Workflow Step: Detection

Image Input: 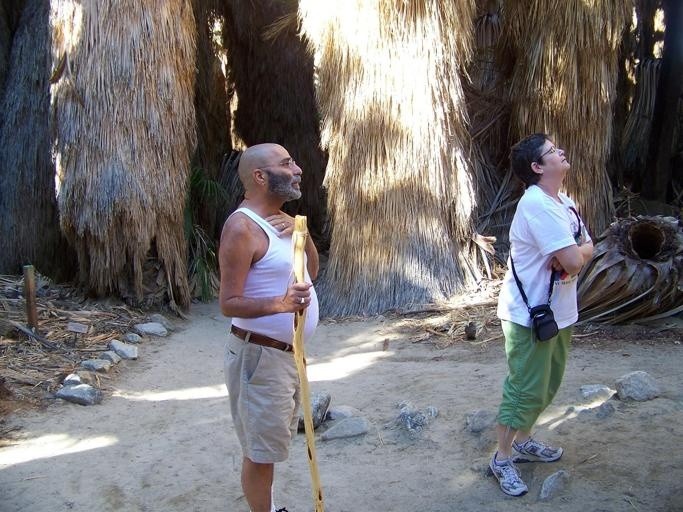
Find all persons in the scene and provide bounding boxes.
[488,131,595,497]
[216,143,320,511]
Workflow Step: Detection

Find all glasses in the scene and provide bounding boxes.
[256,160,295,170]
[537,145,555,161]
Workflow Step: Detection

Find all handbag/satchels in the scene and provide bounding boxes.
[530,305,558,341]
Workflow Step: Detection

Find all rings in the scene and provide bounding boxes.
[299,296,305,307]
[280,221,286,227]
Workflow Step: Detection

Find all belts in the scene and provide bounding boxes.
[230,324,293,352]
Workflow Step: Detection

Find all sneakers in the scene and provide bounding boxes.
[511,436,563,462]
[488,450,528,496]
